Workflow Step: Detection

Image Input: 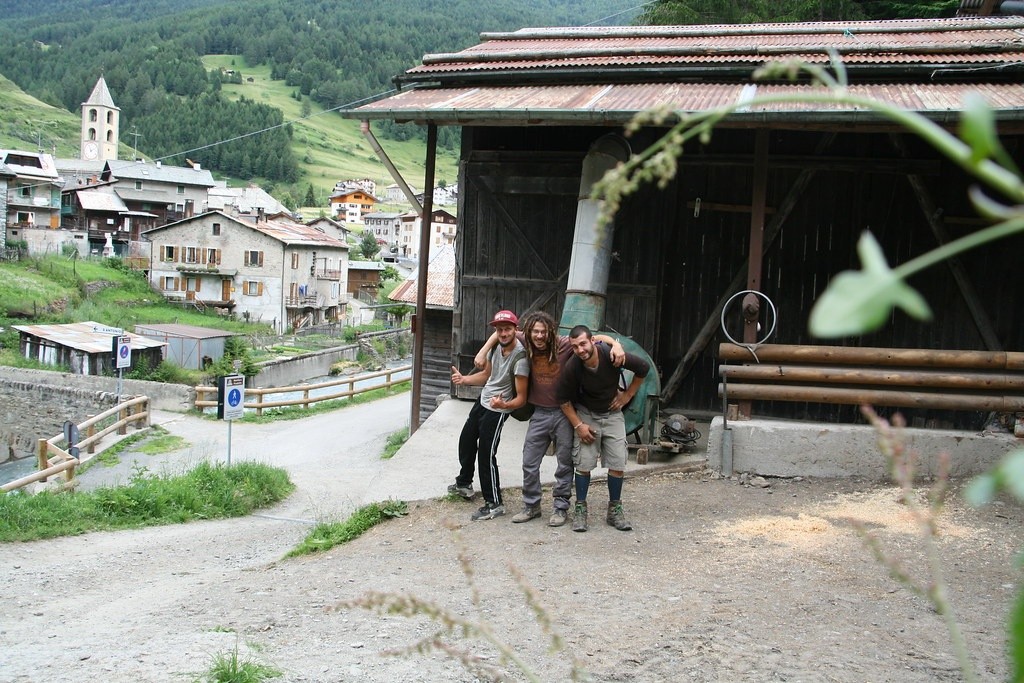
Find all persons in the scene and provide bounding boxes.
[447,310,650,532]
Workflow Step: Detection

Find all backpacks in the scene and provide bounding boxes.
[489,341,535,422]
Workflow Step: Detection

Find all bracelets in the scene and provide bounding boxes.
[574,422,583,429]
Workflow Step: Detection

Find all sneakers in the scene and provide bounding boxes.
[471,501,506,521]
[447,483,474,498]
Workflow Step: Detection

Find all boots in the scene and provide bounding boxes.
[572,501,587,531]
[606,500,632,531]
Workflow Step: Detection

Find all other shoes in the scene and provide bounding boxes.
[512,506,542,523]
[547,508,567,526]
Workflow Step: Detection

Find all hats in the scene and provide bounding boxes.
[489,310,519,326]
